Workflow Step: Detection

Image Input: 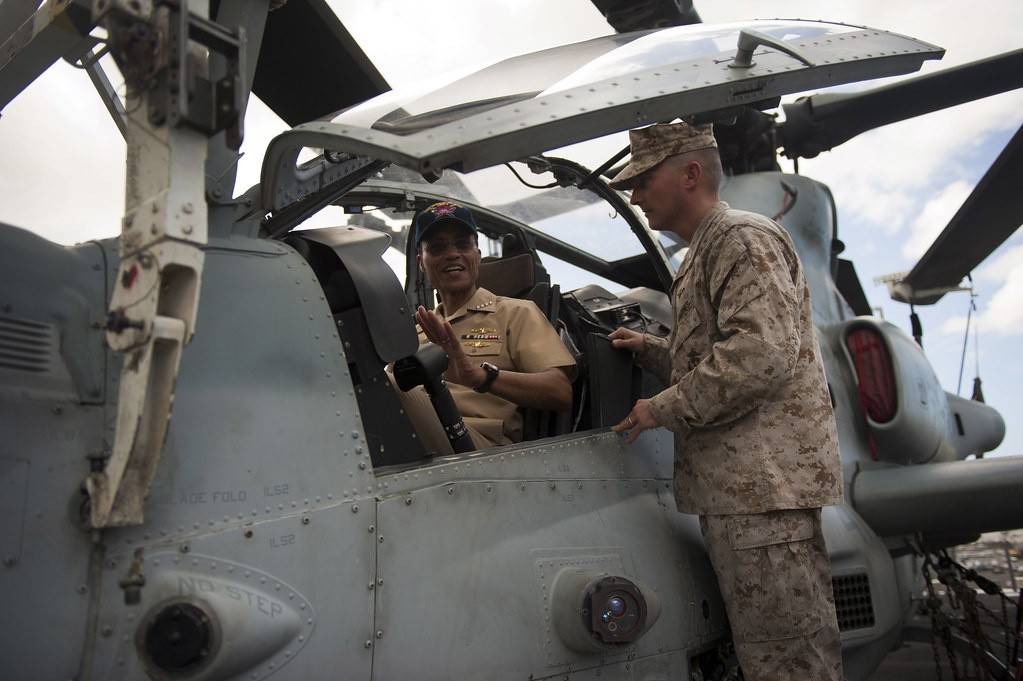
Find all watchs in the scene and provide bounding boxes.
[472,362,499,393]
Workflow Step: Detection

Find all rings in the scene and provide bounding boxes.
[628,418,634,426]
[442,338,450,343]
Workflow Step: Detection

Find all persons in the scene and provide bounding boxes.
[383,203,577,457]
[605,121,850,681]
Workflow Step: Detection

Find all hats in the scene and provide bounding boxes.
[609,121,717,191]
[416,202,477,247]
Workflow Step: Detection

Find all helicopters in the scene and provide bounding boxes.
[0,0,1023,681]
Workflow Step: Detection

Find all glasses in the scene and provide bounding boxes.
[420,240,478,256]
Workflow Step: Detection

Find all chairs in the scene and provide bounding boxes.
[476,252,589,441]
